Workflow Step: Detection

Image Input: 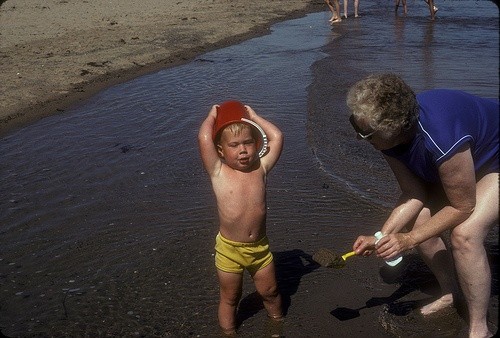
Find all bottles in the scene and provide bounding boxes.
[374,231,403,267]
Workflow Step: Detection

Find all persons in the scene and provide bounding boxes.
[347,70,500,338]
[196,101,285,338]
[325,0,439,25]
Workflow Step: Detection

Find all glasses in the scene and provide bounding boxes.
[349,114,380,140]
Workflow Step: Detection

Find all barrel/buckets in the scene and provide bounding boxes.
[212,100,268,161]
